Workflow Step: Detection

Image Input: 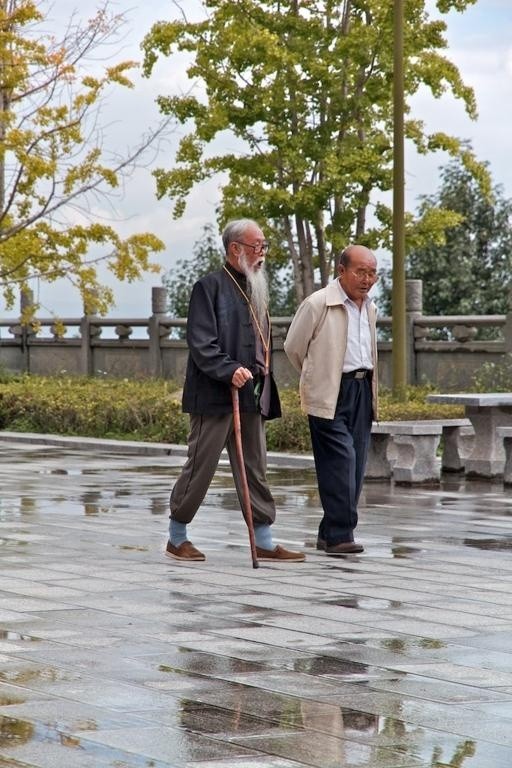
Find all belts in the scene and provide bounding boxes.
[343,371,373,379]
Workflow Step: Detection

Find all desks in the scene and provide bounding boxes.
[426,393,512,483]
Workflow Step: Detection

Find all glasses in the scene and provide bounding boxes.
[236,240,269,255]
[350,270,378,284]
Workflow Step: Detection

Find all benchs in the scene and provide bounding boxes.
[364,418,512,488]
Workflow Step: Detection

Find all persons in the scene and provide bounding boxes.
[165,221,309,562]
[284,245,380,555]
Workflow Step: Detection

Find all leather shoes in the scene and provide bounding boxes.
[318,537,365,554]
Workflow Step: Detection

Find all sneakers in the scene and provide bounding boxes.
[256,544,304,562]
[164,540,205,561]
